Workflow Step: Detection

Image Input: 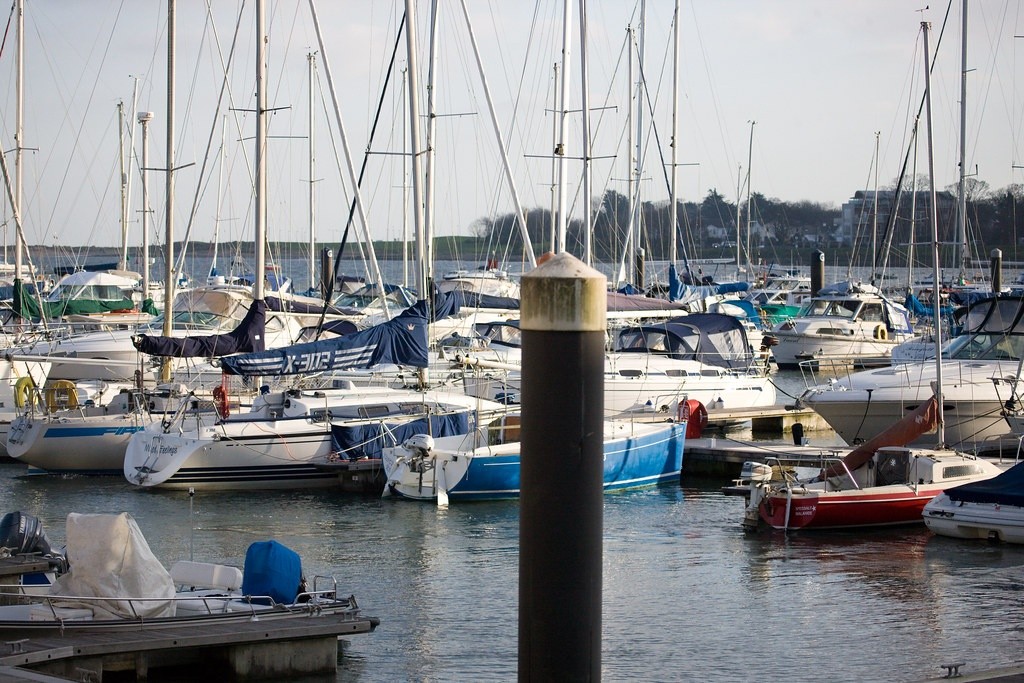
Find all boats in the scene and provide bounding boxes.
[2,510,353,624]
[721,445,1005,532]
[921,460,1024,547]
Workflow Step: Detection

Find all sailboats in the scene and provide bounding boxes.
[1,0,1024,498]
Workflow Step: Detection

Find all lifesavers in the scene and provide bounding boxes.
[42,376,81,414]
[11,375,39,410]
[875,324,889,340]
